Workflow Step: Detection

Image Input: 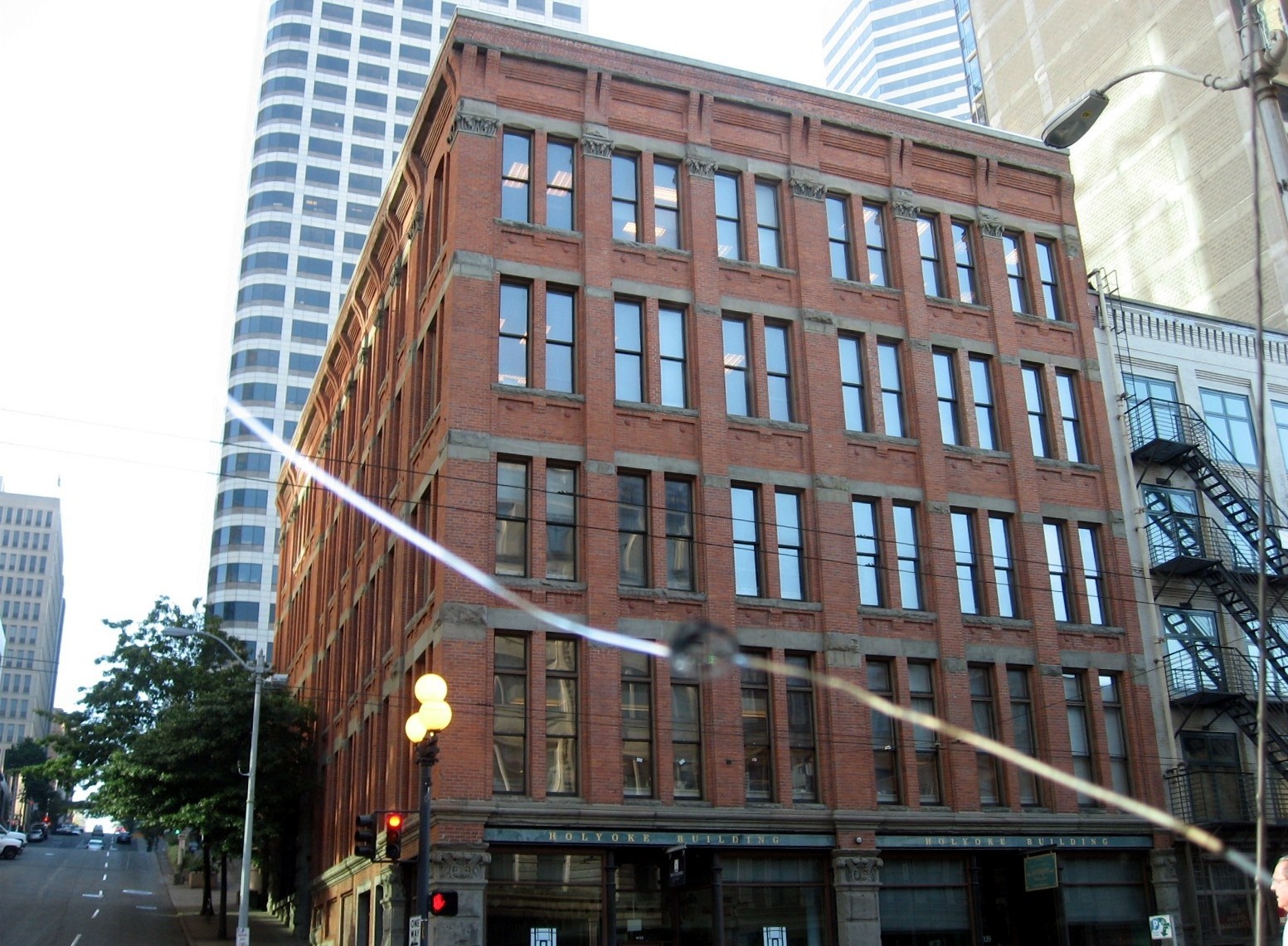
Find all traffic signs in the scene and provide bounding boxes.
[409,915,422,946]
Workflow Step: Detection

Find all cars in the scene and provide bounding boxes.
[56,824,84,835]
[85,839,104,851]
[29,830,43,841]
[116,832,131,843]
[92,827,103,836]
[0,825,28,859]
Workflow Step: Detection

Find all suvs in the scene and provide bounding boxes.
[32,823,47,839]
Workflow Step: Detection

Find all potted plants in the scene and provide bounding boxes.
[249,857,263,892]
[187,854,219,887]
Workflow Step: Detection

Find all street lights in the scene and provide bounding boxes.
[161,627,271,946]
[1037,1,1287,223]
[405,673,452,946]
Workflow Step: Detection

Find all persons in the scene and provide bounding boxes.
[189,839,198,853]
[1270,855,1288,946]
[147,838,154,852]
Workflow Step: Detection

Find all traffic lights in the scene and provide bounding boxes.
[354,815,377,859]
[430,889,459,917]
[386,814,402,859]
[173,828,181,836]
